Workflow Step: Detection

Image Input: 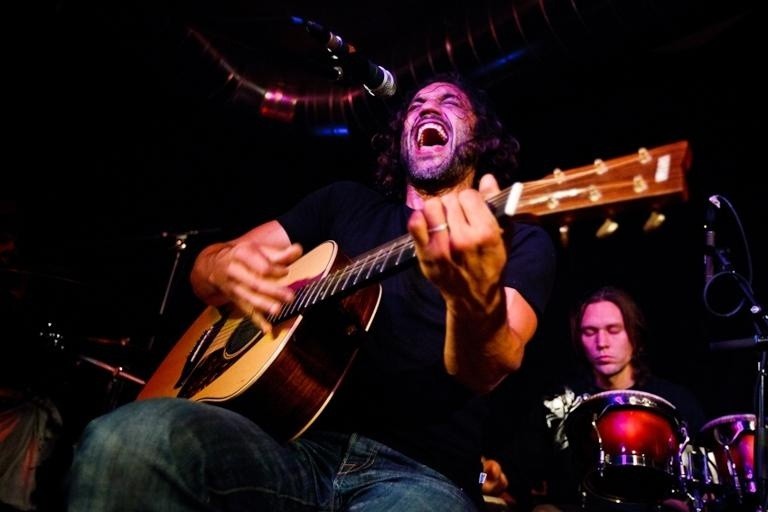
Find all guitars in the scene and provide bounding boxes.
[135,141,691,443]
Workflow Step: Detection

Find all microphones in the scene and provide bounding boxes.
[297,18,398,98]
[703,205,715,284]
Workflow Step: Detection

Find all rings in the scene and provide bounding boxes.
[428,222,449,236]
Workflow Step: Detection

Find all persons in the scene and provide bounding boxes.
[60,73,556,512]
[481,284,707,512]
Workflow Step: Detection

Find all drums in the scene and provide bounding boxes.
[557,390,676,504]
[679,414,758,502]
